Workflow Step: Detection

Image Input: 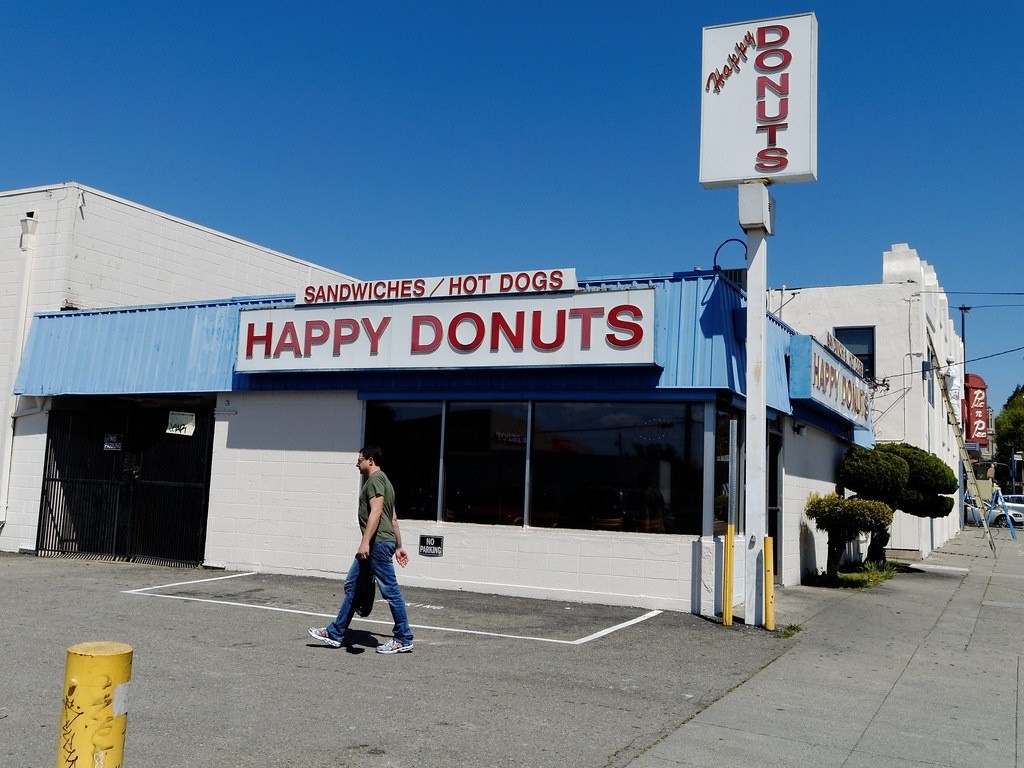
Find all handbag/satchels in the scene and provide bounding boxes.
[354,551,377,617]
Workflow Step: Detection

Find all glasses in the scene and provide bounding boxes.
[358,457,365,462]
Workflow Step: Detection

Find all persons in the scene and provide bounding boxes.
[307,446,416,655]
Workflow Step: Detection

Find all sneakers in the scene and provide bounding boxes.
[308,627,342,647]
[377,638,414,654]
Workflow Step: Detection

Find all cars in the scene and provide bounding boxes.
[998,494,1024,513]
[964,498,1024,527]
